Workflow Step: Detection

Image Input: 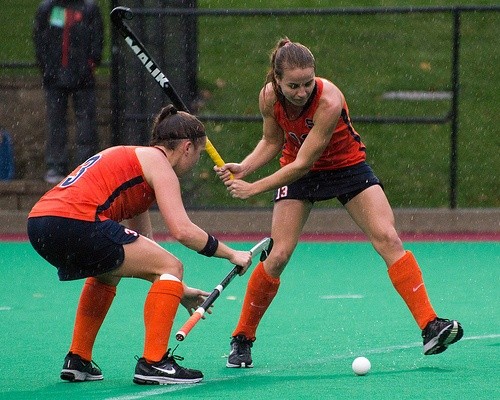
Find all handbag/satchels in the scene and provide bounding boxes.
[0,134,14,181]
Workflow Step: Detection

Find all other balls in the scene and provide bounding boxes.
[351,357,372,376]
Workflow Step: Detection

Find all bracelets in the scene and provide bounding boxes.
[197,234,219,258]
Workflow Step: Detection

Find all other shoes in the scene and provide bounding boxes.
[43,164,68,183]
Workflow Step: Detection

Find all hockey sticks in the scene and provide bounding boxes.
[175,236,275,342]
[108,6,236,193]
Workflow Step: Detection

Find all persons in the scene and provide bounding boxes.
[25,104,252,387]
[214,35,464,369]
[31,0,104,184]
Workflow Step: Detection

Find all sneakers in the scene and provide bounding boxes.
[420,317,464,356]
[59,351,103,381]
[226,334,257,368]
[131,343,204,385]
